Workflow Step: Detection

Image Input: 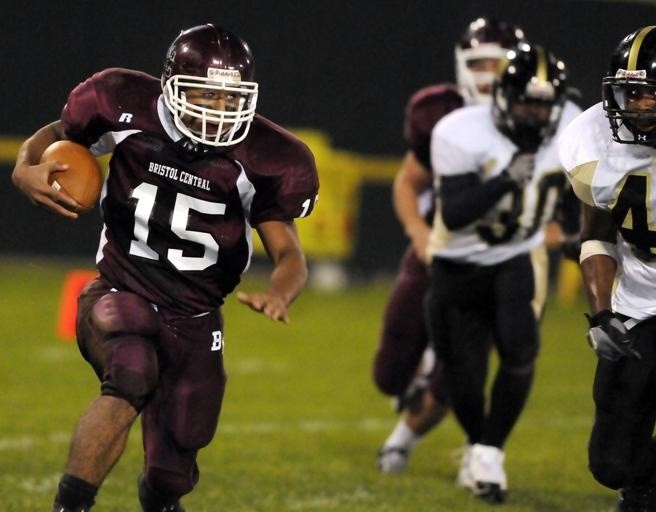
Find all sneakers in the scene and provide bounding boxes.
[374,429,413,479]
[387,343,442,416]
[449,435,510,500]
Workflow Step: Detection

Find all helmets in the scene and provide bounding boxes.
[600,24,656,148]
[157,21,261,160]
[490,41,573,153]
[449,14,528,102]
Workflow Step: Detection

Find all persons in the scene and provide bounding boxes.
[7,20,318,512]
[425,44,583,504]
[366,14,531,478]
[556,28,656,512]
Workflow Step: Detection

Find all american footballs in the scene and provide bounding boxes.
[39,141,103,214]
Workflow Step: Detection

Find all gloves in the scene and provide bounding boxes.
[582,307,644,368]
